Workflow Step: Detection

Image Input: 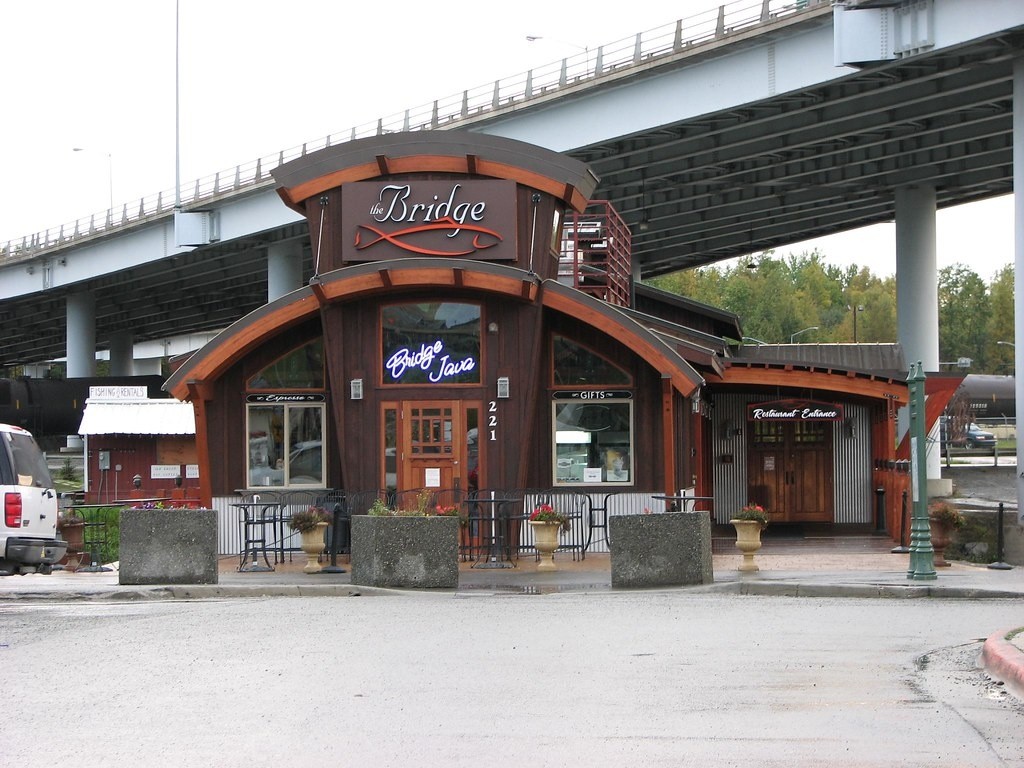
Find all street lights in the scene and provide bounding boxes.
[72,147,113,226]
[853,304,864,343]
[526,35,589,79]
[790,326,819,344]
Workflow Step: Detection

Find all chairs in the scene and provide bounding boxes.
[239,489,587,563]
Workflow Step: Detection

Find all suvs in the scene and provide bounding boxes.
[0,423,69,577]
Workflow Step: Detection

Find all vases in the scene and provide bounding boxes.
[350,514,459,588]
[528,519,561,571]
[120,506,219,585]
[609,511,714,591]
[295,522,329,574]
[730,520,768,571]
[928,518,952,567]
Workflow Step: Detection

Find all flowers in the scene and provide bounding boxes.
[369,487,471,529]
[125,501,208,509]
[529,504,571,536]
[731,501,769,531]
[928,500,968,533]
[643,507,654,514]
[286,506,332,534]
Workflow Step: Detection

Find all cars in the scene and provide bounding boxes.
[939,415,998,450]
[249,439,322,487]
[385,444,441,488]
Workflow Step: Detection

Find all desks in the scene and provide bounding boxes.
[228,502,281,572]
[63,503,125,572]
[652,496,714,512]
[464,499,522,568]
